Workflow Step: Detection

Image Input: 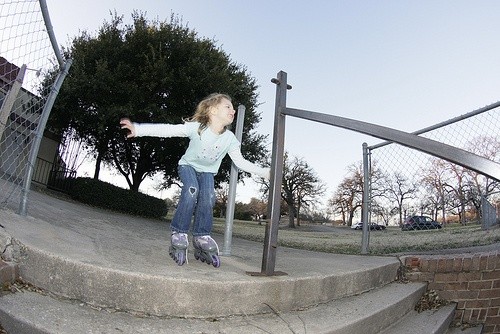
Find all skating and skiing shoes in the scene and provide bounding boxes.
[169,231,189,265]
[192,235,220,268]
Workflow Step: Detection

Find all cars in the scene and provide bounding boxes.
[351,222,387,231]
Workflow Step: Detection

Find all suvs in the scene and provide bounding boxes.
[402,216,443,231]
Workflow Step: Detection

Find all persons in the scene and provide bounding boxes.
[120,94,270,251]
